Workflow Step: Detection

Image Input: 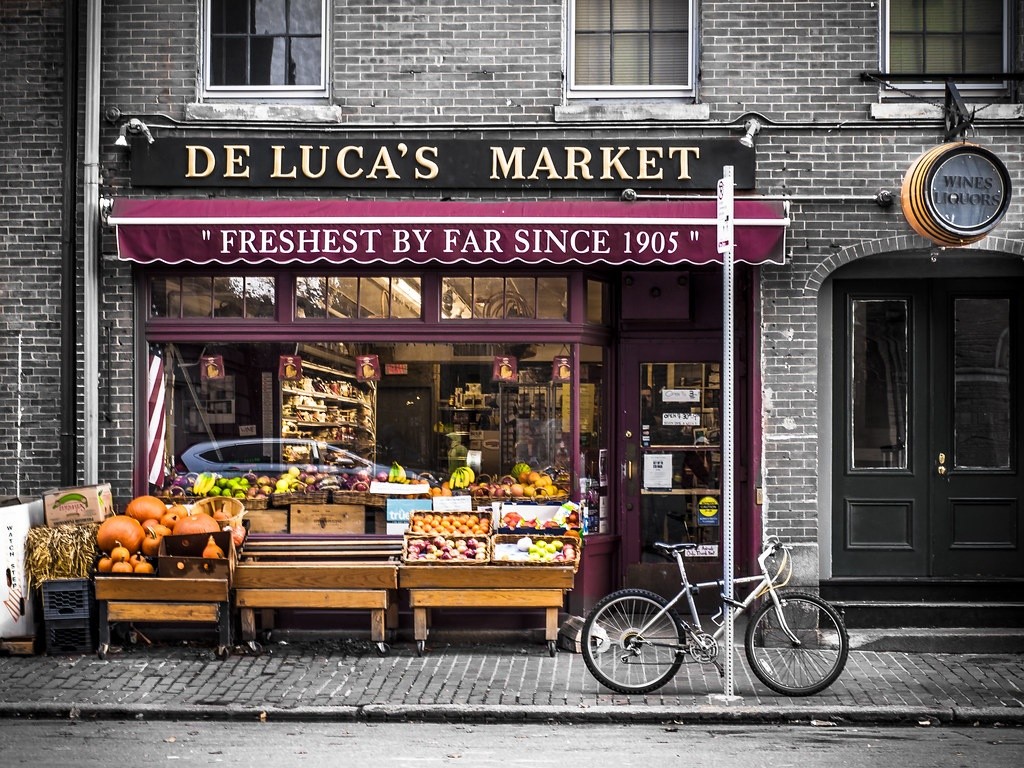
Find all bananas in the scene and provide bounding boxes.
[193,472,215,497]
[449,466,475,490]
[388,461,406,483]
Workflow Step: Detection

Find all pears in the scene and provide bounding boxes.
[207,477,250,498]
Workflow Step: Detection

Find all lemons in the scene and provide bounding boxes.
[528,539,563,561]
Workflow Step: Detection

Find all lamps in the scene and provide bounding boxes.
[114,123,131,146]
[738,118,761,148]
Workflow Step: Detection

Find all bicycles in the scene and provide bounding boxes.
[581,534,849,698]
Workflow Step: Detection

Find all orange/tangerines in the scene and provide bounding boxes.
[402,479,453,500]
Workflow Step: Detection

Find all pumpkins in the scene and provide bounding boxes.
[96,496,246,574]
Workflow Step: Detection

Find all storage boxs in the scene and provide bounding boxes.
[431,495,472,513]
[386,498,433,536]
[290,504,365,535]
[498,501,582,538]
[244,510,289,533]
[41,578,95,620]
[43,483,112,529]
[0,499,44,638]
[91,554,158,577]
[45,618,92,656]
[158,531,238,590]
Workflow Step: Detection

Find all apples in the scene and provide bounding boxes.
[246,473,277,499]
[408,536,486,562]
[411,514,489,536]
[520,472,563,496]
[291,470,373,492]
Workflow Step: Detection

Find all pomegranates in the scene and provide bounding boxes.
[469,480,525,497]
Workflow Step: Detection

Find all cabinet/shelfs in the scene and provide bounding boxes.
[279,359,377,478]
[641,363,722,545]
[182,376,236,450]
[438,382,603,476]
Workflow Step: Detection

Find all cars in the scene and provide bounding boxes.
[175,437,441,493]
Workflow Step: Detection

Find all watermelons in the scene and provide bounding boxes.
[512,463,531,478]
